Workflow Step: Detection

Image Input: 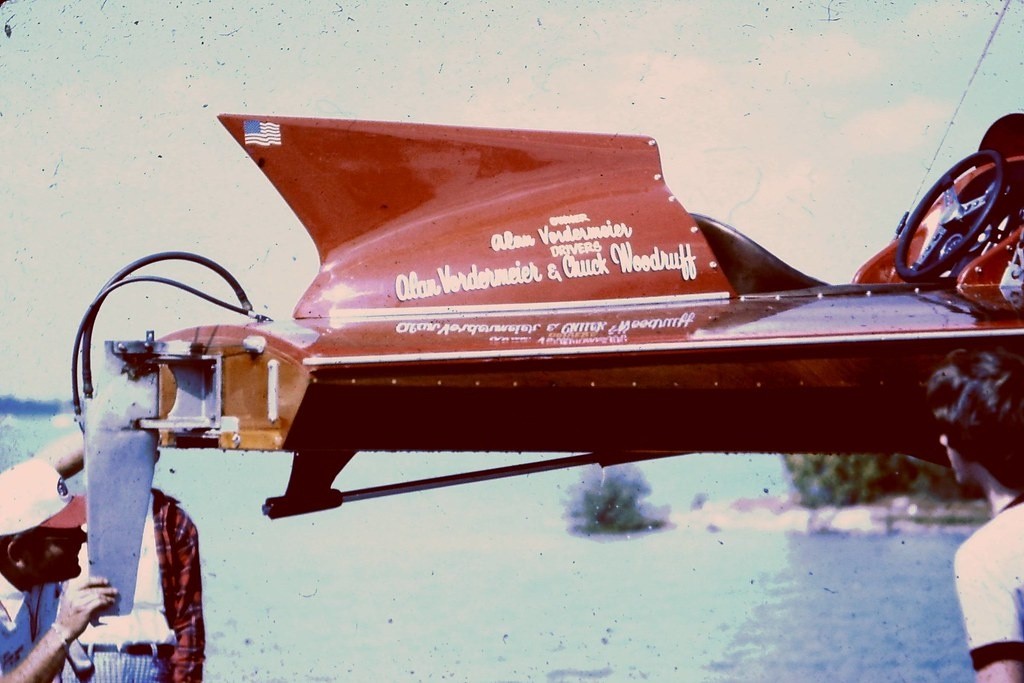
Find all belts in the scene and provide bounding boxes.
[81,644,175,659]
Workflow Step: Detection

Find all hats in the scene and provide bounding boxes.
[0,458,88,536]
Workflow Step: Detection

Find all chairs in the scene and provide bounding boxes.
[689,212,826,292]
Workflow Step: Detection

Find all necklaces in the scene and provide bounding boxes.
[24,584,42,643]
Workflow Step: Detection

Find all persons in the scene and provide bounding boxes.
[928,342,1024,683]
[0,458,119,683]
[62,430,207,683]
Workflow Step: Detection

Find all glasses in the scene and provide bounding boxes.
[28,525,81,545]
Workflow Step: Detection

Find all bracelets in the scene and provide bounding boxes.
[52,624,67,646]
